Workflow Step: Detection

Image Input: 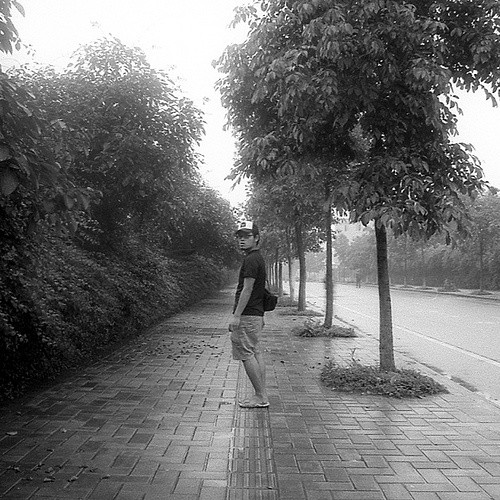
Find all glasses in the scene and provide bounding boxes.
[236,234,254,238]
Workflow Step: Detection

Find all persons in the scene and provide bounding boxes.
[356,270,362,288]
[228,221,270,407]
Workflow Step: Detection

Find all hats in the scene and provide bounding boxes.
[235,221,258,235]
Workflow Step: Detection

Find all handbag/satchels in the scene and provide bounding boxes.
[263,288,278,311]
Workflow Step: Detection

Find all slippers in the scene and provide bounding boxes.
[239,398,270,407]
